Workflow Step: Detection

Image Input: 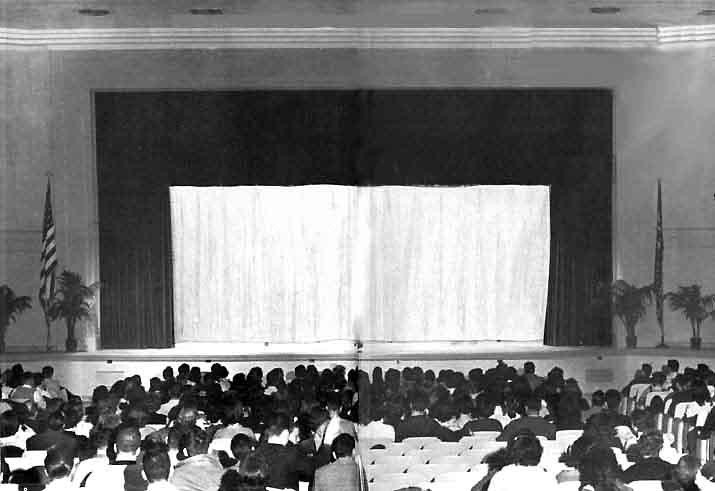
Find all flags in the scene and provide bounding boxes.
[38,185,58,321]
[653,197,664,327]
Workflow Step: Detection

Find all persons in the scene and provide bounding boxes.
[622,359,713,453]
[472,427,544,489]
[564,389,712,488]
[1,363,96,489]
[87,362,588,443]
[80,410,360,488]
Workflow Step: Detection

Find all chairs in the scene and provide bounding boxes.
[0,377,714,491]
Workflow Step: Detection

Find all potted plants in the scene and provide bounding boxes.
[49,270,106,353]
[591,276,663,348]
[1,283,36,352]
[662,283,715,349]
